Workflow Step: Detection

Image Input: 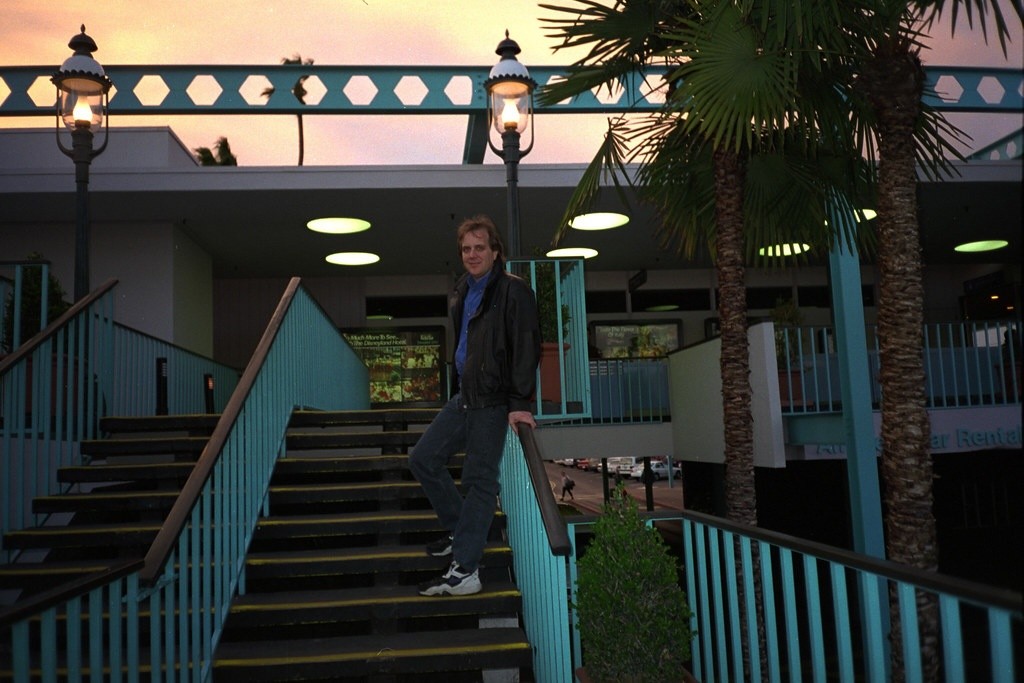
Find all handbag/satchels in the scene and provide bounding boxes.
[564,476,575,489]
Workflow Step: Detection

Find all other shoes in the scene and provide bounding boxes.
[572,497,574,499]
[560,498,564,501]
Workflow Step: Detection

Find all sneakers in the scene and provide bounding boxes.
[417,558,484,599]
[426,532,457,560]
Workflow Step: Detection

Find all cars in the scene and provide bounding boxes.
[557,454,644,478]
[630,461,683,482]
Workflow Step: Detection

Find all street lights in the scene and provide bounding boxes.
[482,29,539,287]
[48,23,115,312]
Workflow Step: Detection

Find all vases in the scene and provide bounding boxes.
[565,478,700,683]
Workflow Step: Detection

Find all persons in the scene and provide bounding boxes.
[615,466,621,486]
[560,471,574,501]
[407,216,543,597]
